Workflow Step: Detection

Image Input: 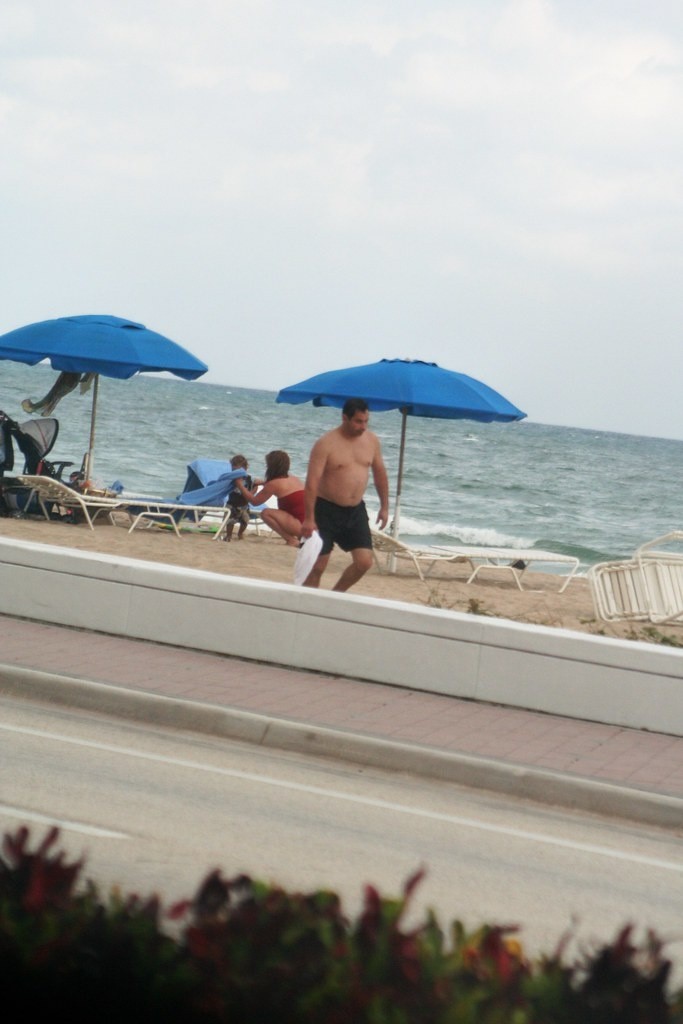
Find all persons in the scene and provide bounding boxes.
[299,397,390,592]
[223,451,307,547]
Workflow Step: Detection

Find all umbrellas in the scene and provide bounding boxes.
[0,315,208,484]
[275,358,528,576]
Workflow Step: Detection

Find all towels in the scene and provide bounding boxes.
[291,530,323,586]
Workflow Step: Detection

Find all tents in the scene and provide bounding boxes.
[175,460,269,517]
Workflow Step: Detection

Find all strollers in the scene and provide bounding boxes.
[0,409,86,516]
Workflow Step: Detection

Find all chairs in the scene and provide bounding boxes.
[588,531,683,627]
[15,459,271,540]
[366,525,580,593]
